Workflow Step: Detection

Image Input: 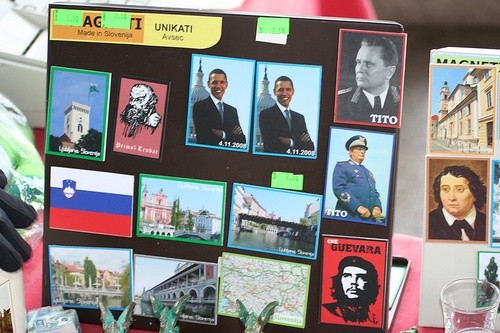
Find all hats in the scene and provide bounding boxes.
[346,135,367,150]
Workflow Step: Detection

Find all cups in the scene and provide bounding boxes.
[441,278,500,333]
[455,327,497,333]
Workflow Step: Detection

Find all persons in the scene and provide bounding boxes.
[429,165,487,241]
[193,69,246,146]
[338,36,400,124]
[332,135,382,218]
[259,75,314,155]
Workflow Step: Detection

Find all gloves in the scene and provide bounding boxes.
[0,168,38,273]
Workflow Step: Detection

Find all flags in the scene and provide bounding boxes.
[48,166,135,238]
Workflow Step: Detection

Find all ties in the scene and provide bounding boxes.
[285,110,291,125]
[217,102,224,115]
[374,96,382,110]
